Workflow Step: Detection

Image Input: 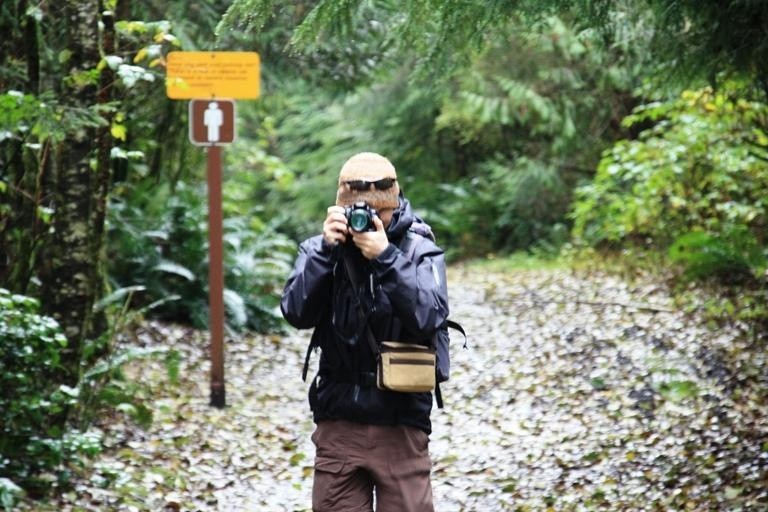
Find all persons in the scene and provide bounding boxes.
[279,151,451,512]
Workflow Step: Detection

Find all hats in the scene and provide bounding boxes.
[336,152,400,209]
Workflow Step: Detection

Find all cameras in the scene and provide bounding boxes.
[337,201,380,232]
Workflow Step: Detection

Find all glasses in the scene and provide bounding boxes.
[345,178,396,191]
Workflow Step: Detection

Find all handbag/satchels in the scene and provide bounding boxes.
[376,341,437,393]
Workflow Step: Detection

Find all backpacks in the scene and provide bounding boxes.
[402,216,450,384]
